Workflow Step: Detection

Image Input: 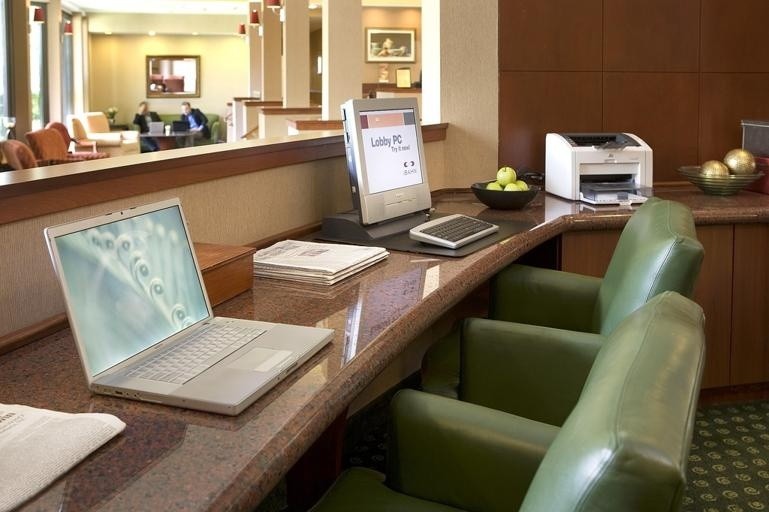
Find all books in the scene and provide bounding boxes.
[252,238,391,286]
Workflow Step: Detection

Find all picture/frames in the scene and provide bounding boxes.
[363,25,416,65]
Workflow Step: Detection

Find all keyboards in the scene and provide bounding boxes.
[409,213,500,249]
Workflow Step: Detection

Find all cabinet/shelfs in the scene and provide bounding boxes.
[510,224,732,388]
[733,221,769,386]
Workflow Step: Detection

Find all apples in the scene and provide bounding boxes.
[496,167,517,187]
[516,180,528,191]
[504,183,522,191]
[486,183,504,191]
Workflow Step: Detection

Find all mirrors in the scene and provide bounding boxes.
[145,54,200,97]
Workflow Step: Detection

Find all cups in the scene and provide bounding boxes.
[165,125,170,133]
[166,131,170,136]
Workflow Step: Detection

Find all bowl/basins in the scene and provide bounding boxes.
[470,183,540,208]
[676,165,766,198]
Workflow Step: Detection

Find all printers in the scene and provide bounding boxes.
[545,132,654,205]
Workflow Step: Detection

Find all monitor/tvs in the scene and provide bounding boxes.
[322,97,432,242]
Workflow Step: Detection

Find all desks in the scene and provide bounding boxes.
[140,131,192,154]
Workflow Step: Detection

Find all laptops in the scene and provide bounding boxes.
[148,122,164,134]
[173,121,186,131]
[44,196,335,417]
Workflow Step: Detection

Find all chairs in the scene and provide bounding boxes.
[0,108,140,173]
[431,200,707,431]
[299,294,708,512]
[151,74,185,92]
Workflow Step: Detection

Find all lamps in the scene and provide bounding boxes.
[247,9,266,39]
[58,19,75,44]
[24,6,45,35]
[262,1,286,21]
[236,21,249,42]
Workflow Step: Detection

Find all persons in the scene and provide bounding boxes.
[175,101,210,148]
[133,101,163,151]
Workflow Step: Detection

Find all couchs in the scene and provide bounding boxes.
[135,112,226,146]
